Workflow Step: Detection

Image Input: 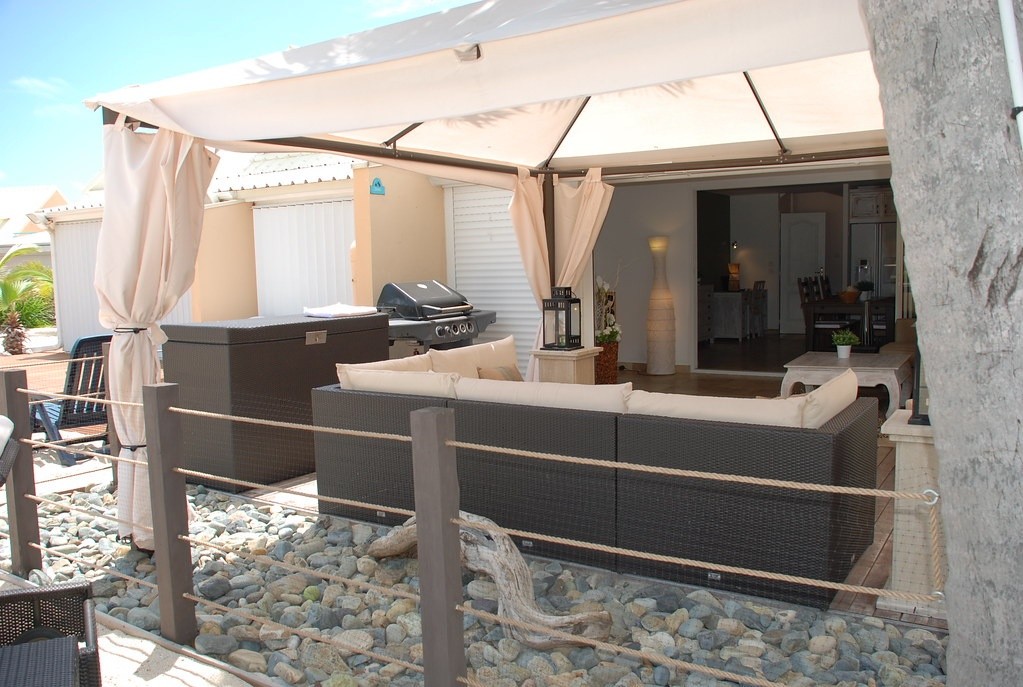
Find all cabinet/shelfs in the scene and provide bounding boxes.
[709,291,766,344]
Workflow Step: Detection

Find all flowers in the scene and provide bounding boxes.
[595,261,624,345]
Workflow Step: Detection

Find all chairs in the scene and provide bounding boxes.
[748,279,766,339]
[26,332,114,467]
[796,273,896,352]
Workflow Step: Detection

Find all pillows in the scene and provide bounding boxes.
[334,335,859,429]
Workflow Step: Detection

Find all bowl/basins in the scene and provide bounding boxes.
[840,291,861,303]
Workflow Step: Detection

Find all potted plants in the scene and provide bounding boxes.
[831,328,861,359]
[854,280,876,301]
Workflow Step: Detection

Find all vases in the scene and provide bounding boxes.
[593,340,620,385]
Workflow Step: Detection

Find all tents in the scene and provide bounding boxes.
[86,1,1022,550]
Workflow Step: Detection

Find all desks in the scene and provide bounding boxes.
[800,295,883,349]
[780,351,913,420]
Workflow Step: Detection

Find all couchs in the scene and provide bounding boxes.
[310,385,880,612]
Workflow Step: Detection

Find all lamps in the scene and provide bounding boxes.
[644,234,676,375]
